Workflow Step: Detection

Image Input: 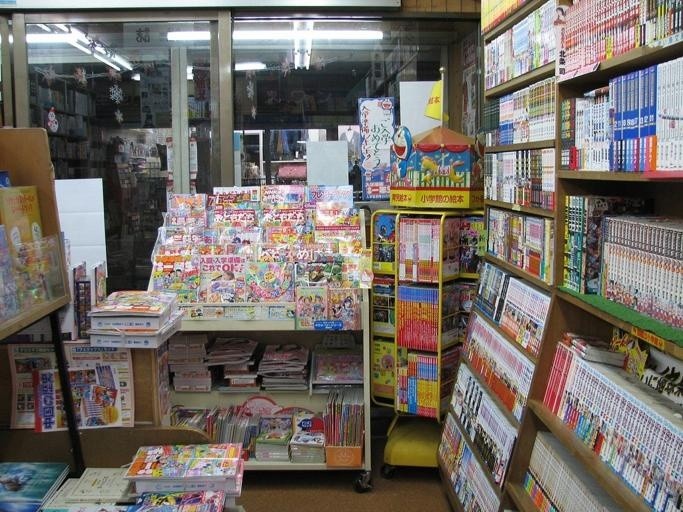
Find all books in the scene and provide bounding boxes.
[29,80,107,180]
[1,171,373,511]
[372,215,486,420]
[441,0,681,508]
